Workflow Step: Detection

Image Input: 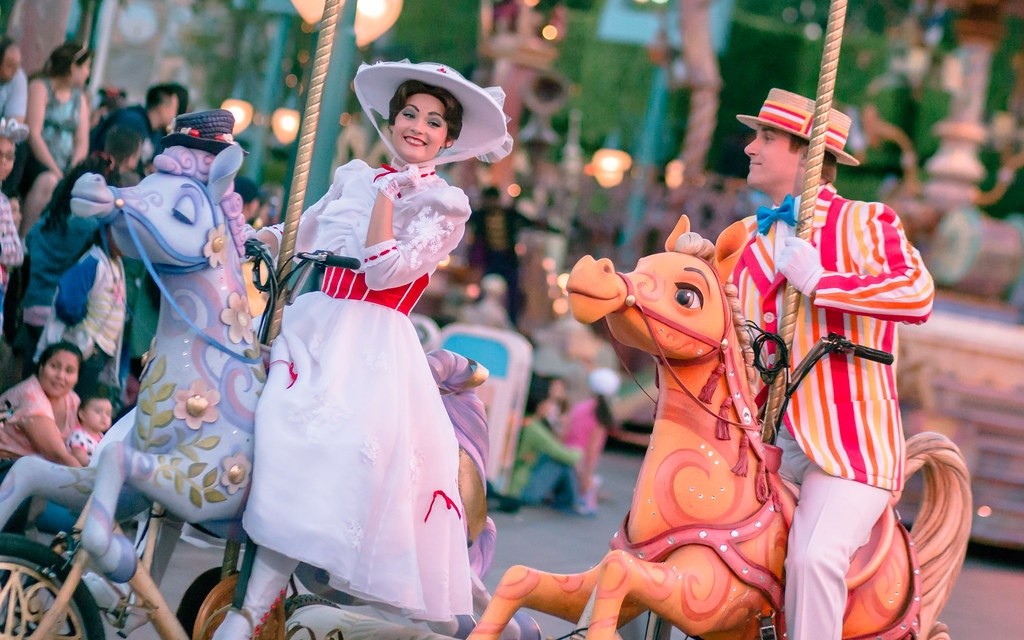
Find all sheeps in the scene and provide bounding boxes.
[465,215,974,640]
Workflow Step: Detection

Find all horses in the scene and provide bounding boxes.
[0,144,542,640]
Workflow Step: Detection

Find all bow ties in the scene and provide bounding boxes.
[754,194,795,235]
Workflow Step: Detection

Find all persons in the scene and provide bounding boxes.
[211,60,514,640]
[468,184,571,329]
[501,366,620,520]
[709,87,934,640]
[0,36,191,544]
[235,173,273,237]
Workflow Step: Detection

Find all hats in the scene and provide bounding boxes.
[163,110,250,155]
[737,88,861,166]
[353,58,514,168]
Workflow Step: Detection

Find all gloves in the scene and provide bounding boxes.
[775,223,825,299]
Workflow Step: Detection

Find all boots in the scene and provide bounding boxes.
[210,609,255,640]
[78,515,182,638]
